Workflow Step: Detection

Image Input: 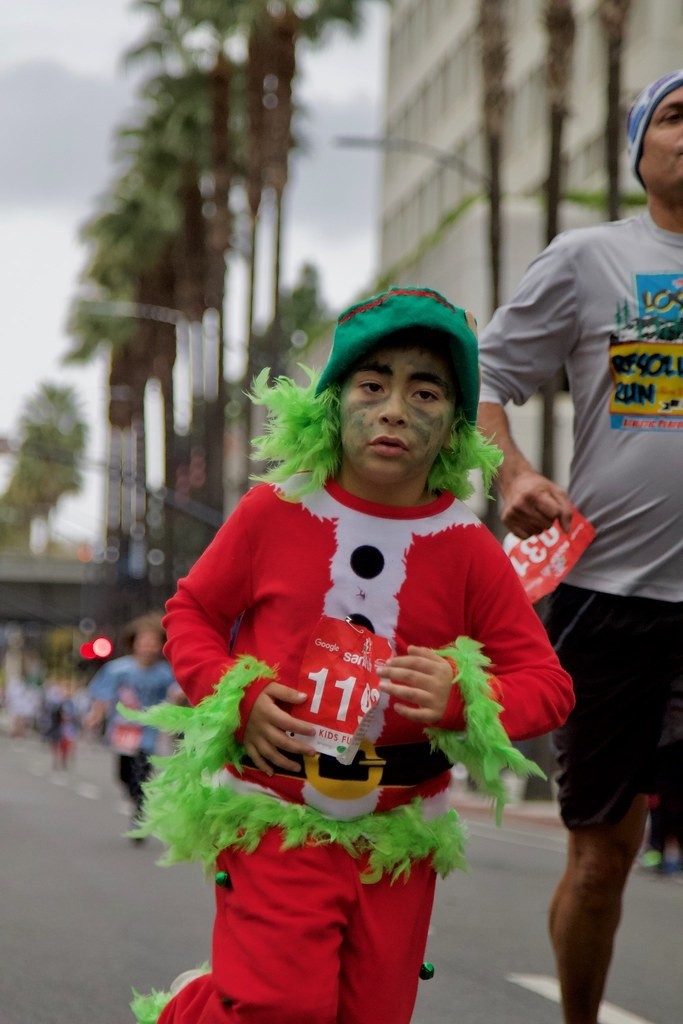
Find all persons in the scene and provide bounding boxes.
[82,627,185,848]
[639,797,682,876]
[133,285,578,1024]
[477,71,683,1024]
[9,676,79,770]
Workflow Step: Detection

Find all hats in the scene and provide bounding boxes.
[627,71,683,191]
[314,287,479,433]
